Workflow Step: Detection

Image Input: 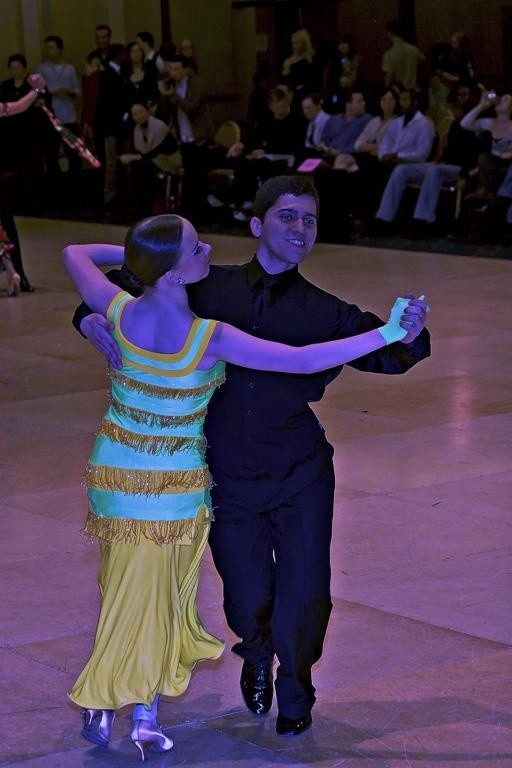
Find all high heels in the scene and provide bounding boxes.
[128,719,176,763]
[80,703,117,749]
[8,272,21,297]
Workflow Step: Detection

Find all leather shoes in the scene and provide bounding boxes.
[19,274,34,292]
[274,707,313,737]
[318,181,512,247]
[238,652,276,717]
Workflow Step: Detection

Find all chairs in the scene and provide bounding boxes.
[159,119,241,222]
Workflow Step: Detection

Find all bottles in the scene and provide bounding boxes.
[486,92,501,105]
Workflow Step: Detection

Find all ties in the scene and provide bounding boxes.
[308,123,317,148]
